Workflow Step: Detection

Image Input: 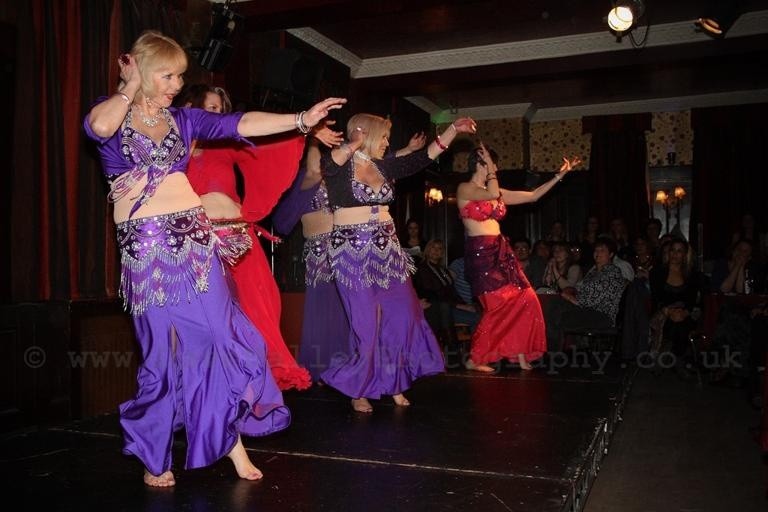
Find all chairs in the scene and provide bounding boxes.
[584,277,641,357]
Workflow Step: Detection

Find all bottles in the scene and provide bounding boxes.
[743,269,752,297]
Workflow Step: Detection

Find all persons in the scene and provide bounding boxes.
[273,130,429,386]
[396,216,480,365]
[174,83,346,392]
[511,213,767,372]
[455,138,581,374]
[317,113,478,414]
[84,29,350,488]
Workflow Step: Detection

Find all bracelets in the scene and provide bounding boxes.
[115,90,132,106]
[294,110,313,135]
[485,172,498,182]
[555,173,563,183]
[435,136,449,151]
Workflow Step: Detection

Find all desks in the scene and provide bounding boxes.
[535,293,583,353]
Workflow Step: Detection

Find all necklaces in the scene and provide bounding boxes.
[139,111,163,128]
[355,150,372,162]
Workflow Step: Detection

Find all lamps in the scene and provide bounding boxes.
[653,186,688,215]
[199,8,239,73]
[606,2,644,42]
[694,0,737,40]
